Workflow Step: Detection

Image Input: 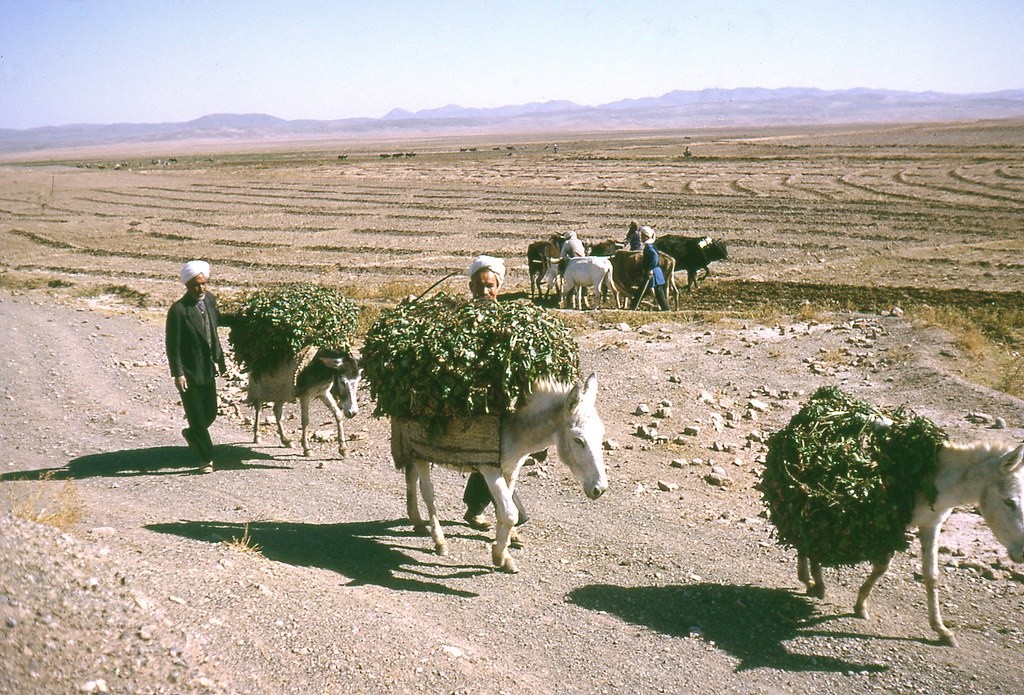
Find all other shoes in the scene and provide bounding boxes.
[198,456,213,474]
[510,530,524,548]
[463,509,495,530]
[182,428,201,454]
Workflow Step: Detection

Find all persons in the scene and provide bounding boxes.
[625,221,641,250]
[462,254,530,548]
[559,231,592,310]
[166,260,238,474]
[626,226,671,311]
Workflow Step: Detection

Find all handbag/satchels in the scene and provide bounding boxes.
[649,267,665,286]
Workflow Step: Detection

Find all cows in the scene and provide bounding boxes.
[76,136,691,161]
[653,234,730,288]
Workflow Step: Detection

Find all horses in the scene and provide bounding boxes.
[246,348,364,459]
[527,229,680,312]
[796,409,1024,648]
[390,372,610,575]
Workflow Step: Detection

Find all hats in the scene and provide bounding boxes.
[468,255,506,290]
[179,260,210,285]
[641,226,655,244]
[564,231,577,239]
